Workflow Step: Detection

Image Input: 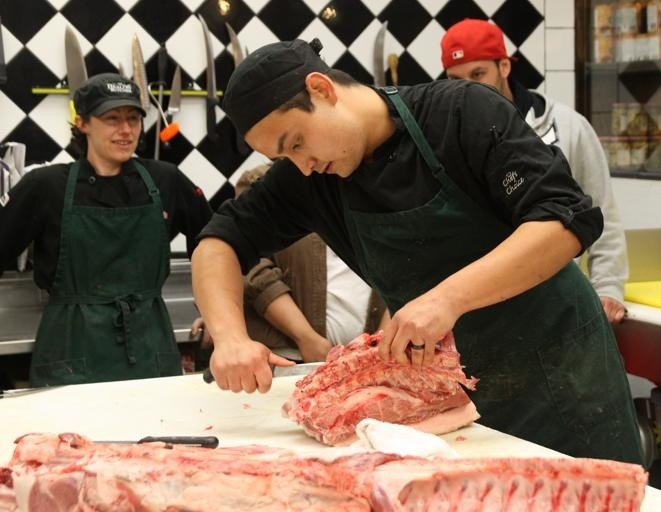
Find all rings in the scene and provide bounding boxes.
[411,343,425,349]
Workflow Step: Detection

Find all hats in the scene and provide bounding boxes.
[441,19,518,69]
[74,74,147,117]
[223,38,329,137]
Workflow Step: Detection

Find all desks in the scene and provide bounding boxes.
[585,234,661,388]
[0,358,660,512]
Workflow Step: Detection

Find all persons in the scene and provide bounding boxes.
[232,155,392,363]
[441,21,629,326]
[189,39,644,469]
[0,74,216,391]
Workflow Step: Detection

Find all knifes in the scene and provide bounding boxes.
[63,12,246,141]
[201,361,330,384]
[91,435,219,449]
[373,21,389,87]
